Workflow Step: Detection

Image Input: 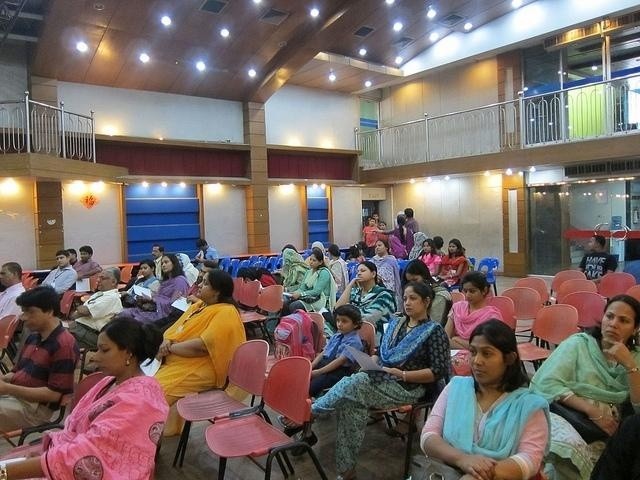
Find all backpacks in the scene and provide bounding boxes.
[274,310,320,362]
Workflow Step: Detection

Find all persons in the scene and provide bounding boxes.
[576,233,615,283]
[40,238,219,350]
[2,286,77,431]
[154,271,247,437]
[276,242,369,328]
[0,317,168,478]
[421,319,552,480]
[441,270,505,377]
[528,293,640,479]
[278,260,453,479]
[2,262,27,319]
[363,207,466,292]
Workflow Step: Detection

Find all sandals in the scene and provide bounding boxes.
[386,421,416,436]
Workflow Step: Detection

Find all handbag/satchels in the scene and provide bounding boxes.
[410,455,461,480]
[549,401,609,444]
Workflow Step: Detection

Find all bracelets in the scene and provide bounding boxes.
[588,408,605,422]
[626,366,639,375]
[2,463,7,478]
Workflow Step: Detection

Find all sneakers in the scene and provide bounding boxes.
[293,432,318,455]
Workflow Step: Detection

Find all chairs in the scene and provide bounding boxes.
[3,245,640,478]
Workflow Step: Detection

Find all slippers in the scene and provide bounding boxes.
[278,416,300,429]
